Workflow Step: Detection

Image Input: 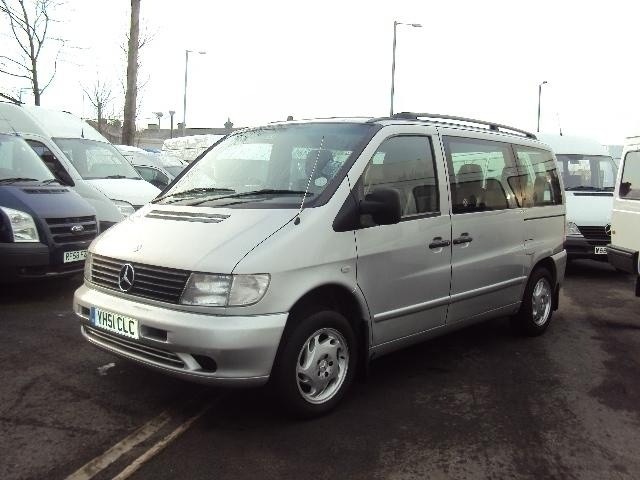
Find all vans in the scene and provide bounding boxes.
[0,96,254,234]
[450,151,621,265]
[604,135,640,296]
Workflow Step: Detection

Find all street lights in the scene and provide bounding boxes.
[182,50,207,122]
[390,19,424,115]
[537,79,553,131]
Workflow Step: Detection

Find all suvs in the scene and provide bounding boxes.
[0,135,101,285]
[71,112,570,415]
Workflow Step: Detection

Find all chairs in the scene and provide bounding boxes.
[455,164,495,208]
[292,150,406,218]
